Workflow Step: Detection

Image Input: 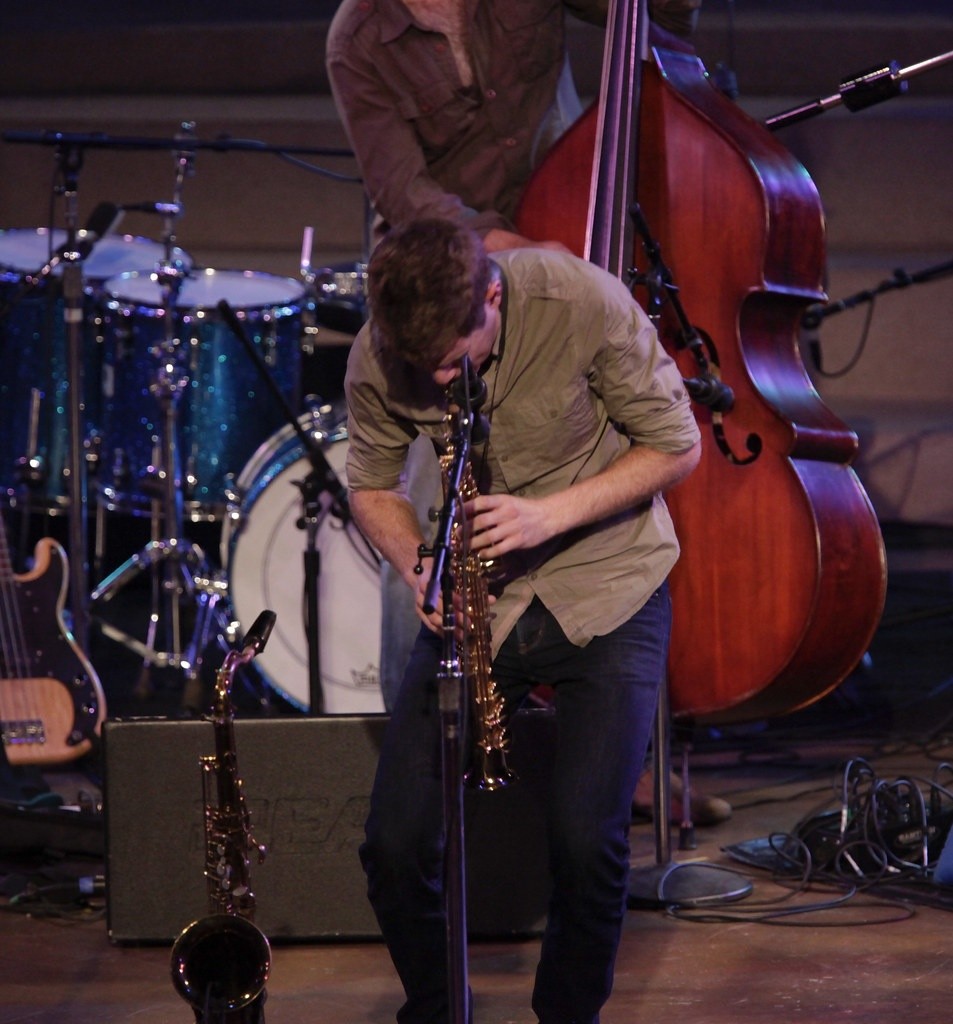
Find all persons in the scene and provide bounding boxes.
[327,0,738,827]
[340,218,702,1024]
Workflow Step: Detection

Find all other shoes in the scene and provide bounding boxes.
[638,772,732,825]
[398,985,477,1024]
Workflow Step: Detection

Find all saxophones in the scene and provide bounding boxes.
[439,380,520,790]
[172,610,279,1024]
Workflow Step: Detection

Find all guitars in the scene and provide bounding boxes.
[0,518,109,763]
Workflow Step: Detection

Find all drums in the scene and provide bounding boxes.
[2,226,189,511]
[221,406,423,717]
[82,268,301,523]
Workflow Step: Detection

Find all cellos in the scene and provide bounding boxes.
[515,3,887,866]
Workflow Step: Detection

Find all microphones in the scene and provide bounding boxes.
[121,199,182,219]
[72,200,119,253]
[682,374,736,414]
[218,297,260,363]
